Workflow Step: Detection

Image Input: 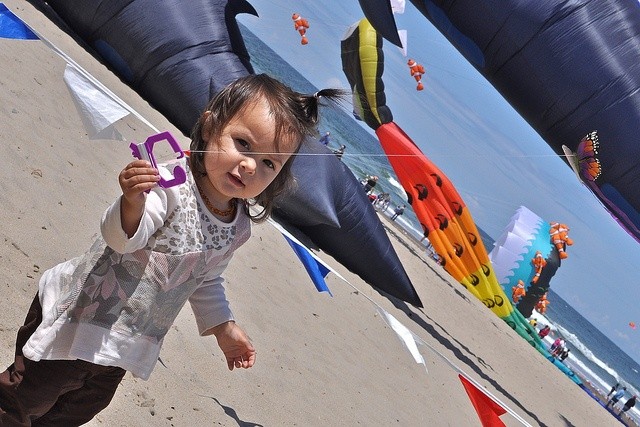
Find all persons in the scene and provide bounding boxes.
[391,205,406,221]
[538,327,550,339]
[529,318,537,326]
[540,325,548,333]
[618,397,636,416]
[550,338,570,362]
[0,73,353,426]
[332,145,346,160]
[394,206,400,212]
[372,193,389,211]
[319,132,330,146]
[364,176,378,193]
[606,387,626,409]
[608,383,619,396]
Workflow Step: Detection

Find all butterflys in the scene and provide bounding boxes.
[560,129,603,185]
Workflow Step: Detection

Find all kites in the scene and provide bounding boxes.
[27,0,425,310]
[549,228,568,258]
[511,280,526,303]
[538,292,550,313]
[408,59,425,90]
[292,12,310,45]
[550,221,574,245]
[561,130,639,244]
[531,249,547,283]
[341,0,640,427]
